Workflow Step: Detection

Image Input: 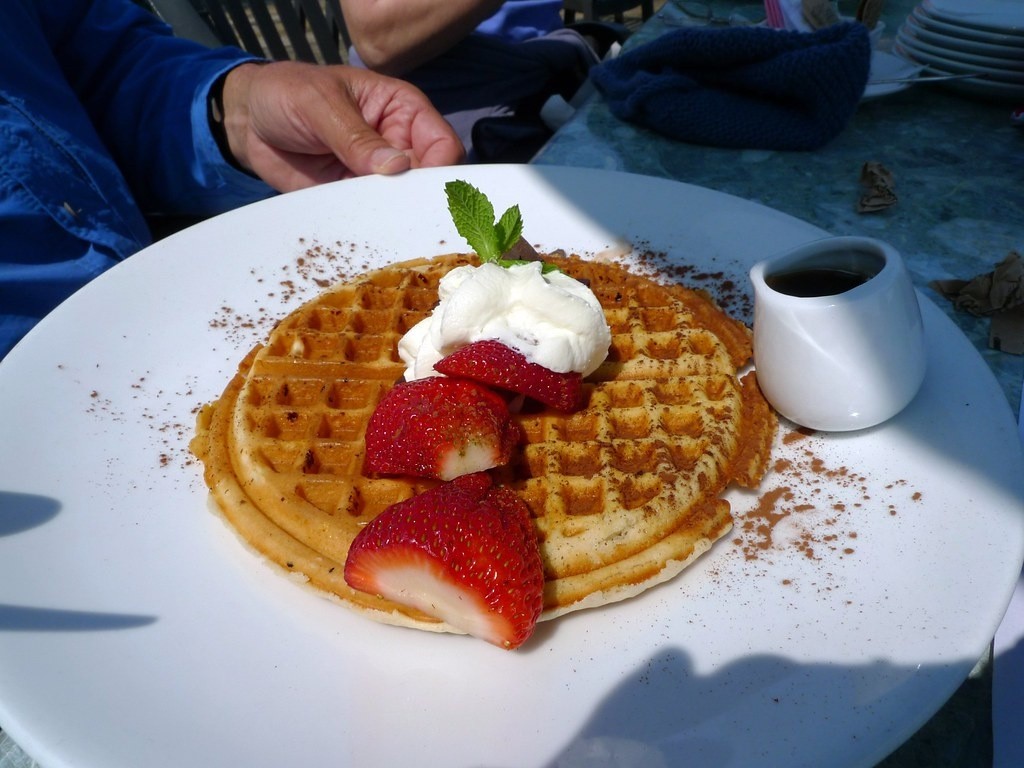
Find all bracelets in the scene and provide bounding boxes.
[206,67,259,179]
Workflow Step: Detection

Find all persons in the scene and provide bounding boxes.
[0,0,633,360]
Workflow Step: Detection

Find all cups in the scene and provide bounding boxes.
[751,237,924,432]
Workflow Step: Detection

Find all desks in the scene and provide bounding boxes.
[524,0,1024,768]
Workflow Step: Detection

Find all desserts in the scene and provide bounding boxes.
[203,178,782,650]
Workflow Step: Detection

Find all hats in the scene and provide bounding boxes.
[590,20,871,152]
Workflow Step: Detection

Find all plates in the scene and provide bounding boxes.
[889,1,1024,99]
[1,166,1022,757]
